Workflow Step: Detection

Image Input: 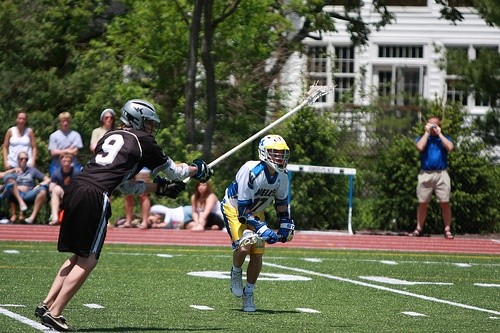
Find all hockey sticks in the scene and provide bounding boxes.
[164,80,336,192]
[236,232,284,249]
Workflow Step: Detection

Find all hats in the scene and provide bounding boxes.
[98,108,117,122]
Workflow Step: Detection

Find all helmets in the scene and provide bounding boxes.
[257,134,291,174]
[120,99,161,138]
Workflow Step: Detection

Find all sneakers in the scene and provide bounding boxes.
[229,266,244,298]
[40,312,77,333]
[34,302,49,317]
[241,287,256,313]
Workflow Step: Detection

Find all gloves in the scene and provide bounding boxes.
[190,159,214,184]
[277,217,294,244]
[254,221,279,244]
[153,174,186,200]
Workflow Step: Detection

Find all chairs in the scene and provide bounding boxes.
[44,155,81,221]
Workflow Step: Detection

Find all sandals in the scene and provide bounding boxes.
[408,229,421,237]
[444,230,454,239]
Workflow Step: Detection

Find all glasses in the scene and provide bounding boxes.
[105,116,113,120]
[19,158,28,162]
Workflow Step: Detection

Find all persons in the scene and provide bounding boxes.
[36,99,215,333]
[221,135,295,312]
[0,109,223,232]
[408,117,456,238]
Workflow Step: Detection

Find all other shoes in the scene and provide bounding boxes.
[117,223,134,228]
[138,224,149,229]
[0,193,62,226]
